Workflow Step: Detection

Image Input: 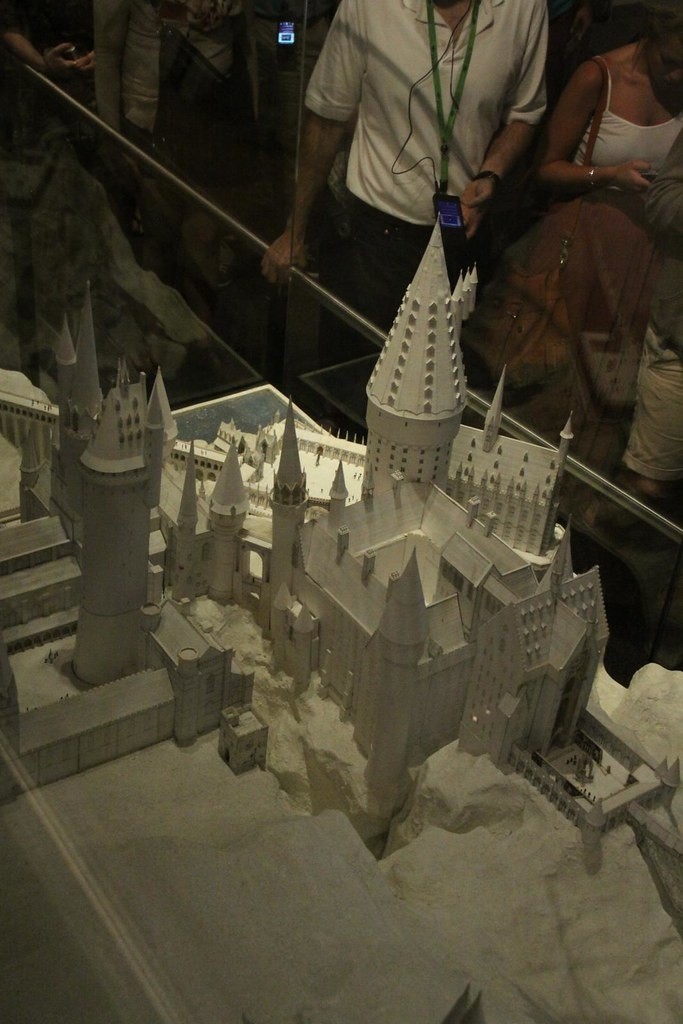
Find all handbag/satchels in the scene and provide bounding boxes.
[457,255,573,388]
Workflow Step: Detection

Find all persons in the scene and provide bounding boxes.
[0,0,682,661]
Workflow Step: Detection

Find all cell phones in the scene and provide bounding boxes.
[277,19,295,44]
[641,173,660,178]
[432,194,466,232]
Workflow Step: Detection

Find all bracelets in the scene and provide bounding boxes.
[589,167,606,187]
[474,171,501,187]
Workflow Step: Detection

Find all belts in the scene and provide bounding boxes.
[253,11,318,25]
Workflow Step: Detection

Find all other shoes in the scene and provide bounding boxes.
[212,237,240,286]
